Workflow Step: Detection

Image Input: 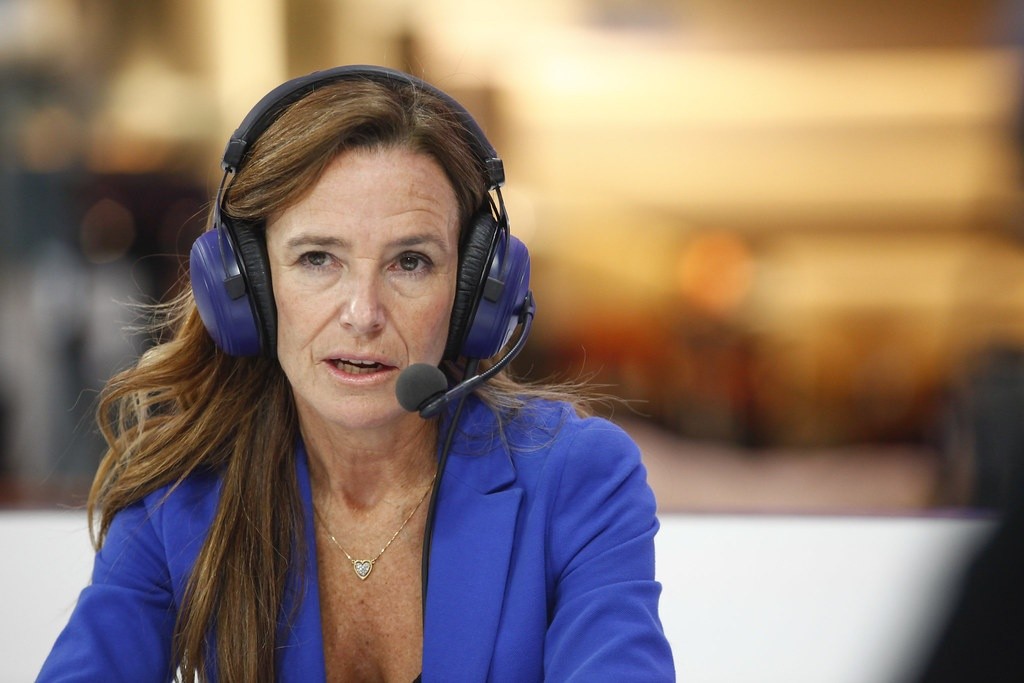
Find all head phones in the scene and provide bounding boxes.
[188,62,531,360]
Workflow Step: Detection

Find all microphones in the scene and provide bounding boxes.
[394,290,535,420]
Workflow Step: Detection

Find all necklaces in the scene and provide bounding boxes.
[313,474,436,581]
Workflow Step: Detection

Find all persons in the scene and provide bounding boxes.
[32,65,676,683]
